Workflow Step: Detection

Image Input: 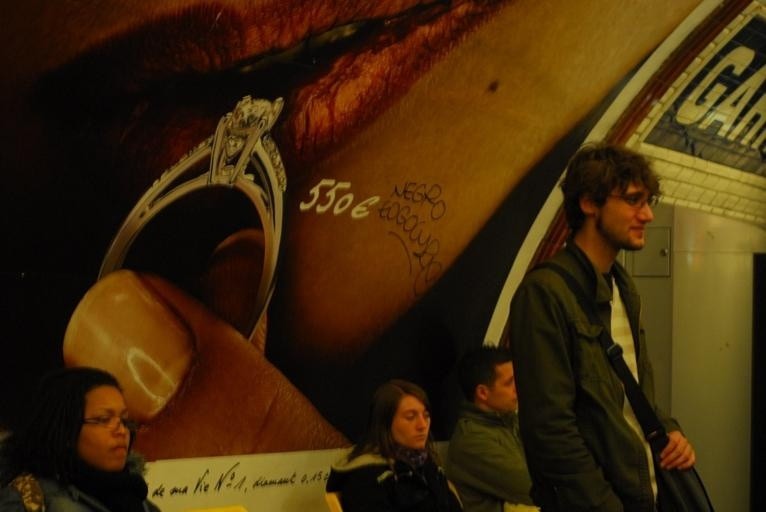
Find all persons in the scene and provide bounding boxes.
[323,380,466,512]
[446,342,540,512]
[508,142,697,511]
[0,0,707,512]
[0,365,162,512]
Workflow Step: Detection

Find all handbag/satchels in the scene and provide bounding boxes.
[653,444,715,511]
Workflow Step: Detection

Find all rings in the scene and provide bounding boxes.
[92,93,290,347]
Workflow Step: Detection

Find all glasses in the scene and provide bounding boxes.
[607,193,659,209]
[81,416,136,434]
